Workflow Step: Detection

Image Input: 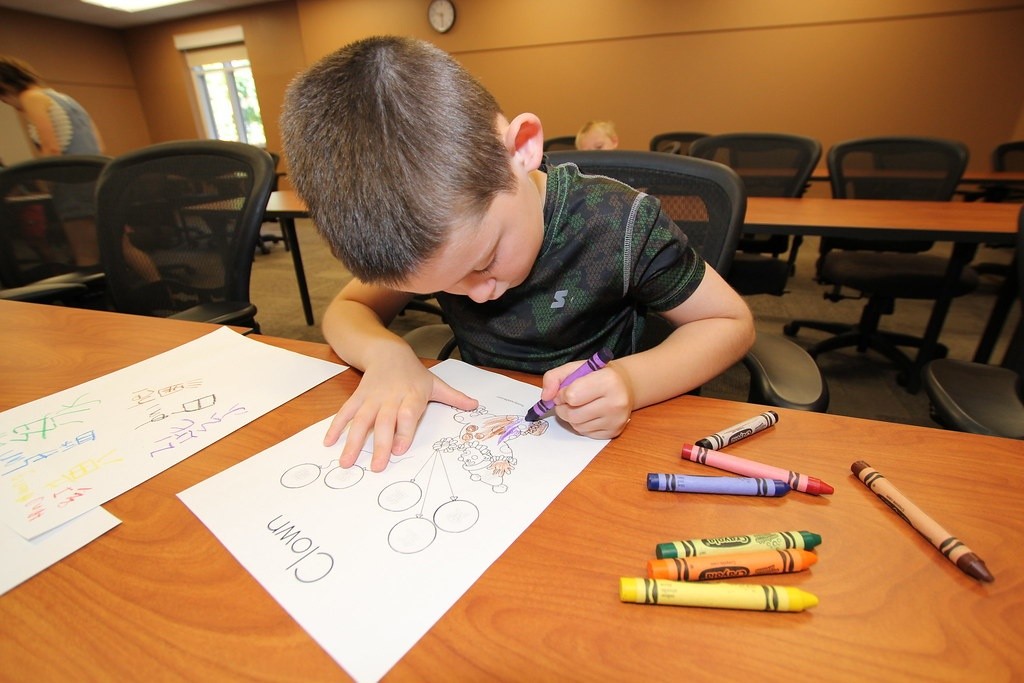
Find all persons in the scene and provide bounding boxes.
[576,120,650,192]
[282,34,756,473]
[0,57,168,299]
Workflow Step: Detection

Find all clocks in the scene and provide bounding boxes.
[429,0,457,32]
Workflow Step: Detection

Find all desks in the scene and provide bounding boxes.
[731,166,1023,203]
[190,192,326,327]
[651,195,1023,396]
[1,297,1023,683]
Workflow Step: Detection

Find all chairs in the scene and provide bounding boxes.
[538,132,1023,437]
[3,133,281,334]
